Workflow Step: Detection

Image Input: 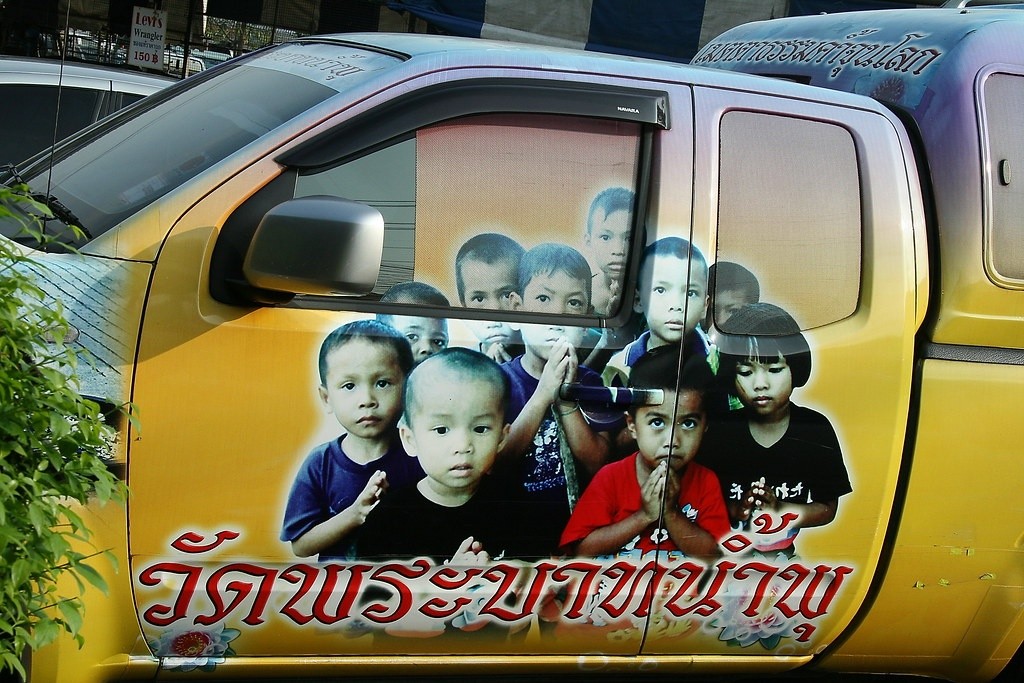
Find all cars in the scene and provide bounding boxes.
[1,54,283,249]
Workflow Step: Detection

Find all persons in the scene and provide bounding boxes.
[279,320,427,562]
[375,185,857,650]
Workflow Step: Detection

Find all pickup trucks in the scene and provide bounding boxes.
[0,1,1023,683]
